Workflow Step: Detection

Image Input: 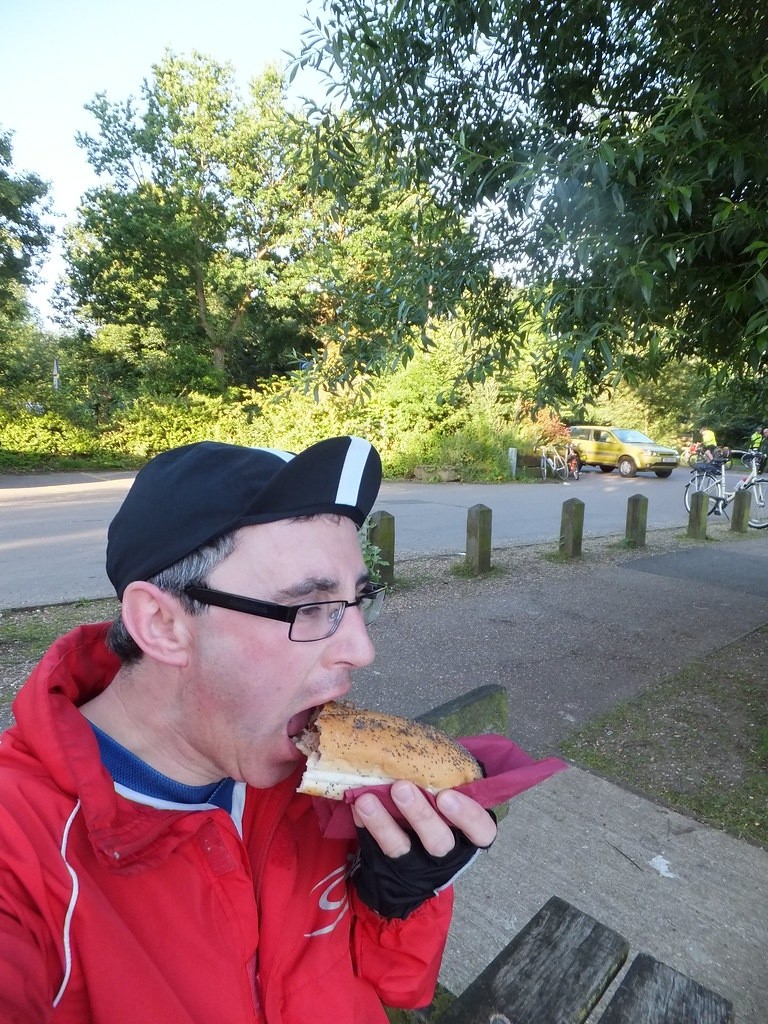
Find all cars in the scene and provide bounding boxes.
[567,426,680,479]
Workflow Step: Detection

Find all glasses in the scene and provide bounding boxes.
[183,580,386,643]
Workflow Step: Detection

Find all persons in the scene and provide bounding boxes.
[750,428,762,450]
[701,427,717,448]
[0,435,498,1023]
[758,429,768,474]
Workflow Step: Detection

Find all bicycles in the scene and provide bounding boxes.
[683,450,768,528]
[533,442,570,481]
[739,449,766,474]
[686,449,733,470]
[561,443,582,480]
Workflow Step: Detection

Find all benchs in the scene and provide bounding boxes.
[432,895,737,1024]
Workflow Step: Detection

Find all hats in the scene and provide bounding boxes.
[104,436,381,602]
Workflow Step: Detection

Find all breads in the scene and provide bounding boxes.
[292,700,485,803]
